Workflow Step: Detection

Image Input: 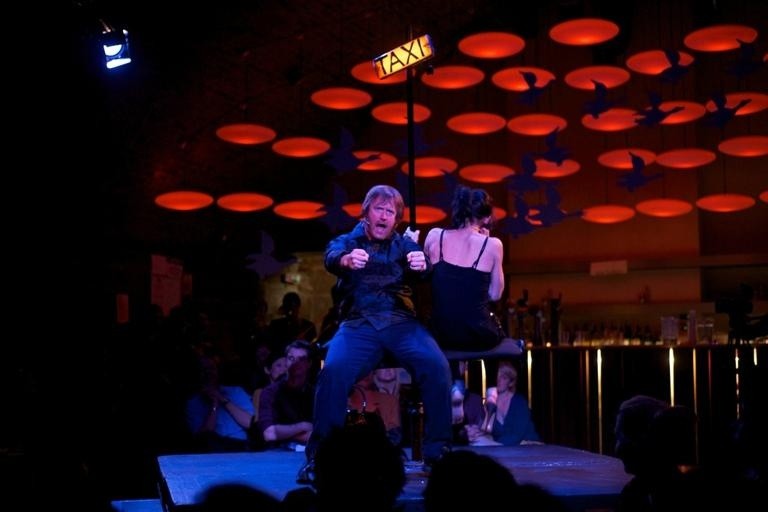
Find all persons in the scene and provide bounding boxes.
[259,340,313,451]
[424,449,564,508]
[351,366,400,444]
[295,182,459,485]
[186,343,256,451]
[402,187,509,354]
[447,359,479,445]
[283,427,407,508]
[252,351,288,422]
[615,395,695,507]
[268,292,317,355]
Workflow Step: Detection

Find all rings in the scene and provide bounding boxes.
[421,266,423,272]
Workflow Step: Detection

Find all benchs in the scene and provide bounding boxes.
[317,337,524,461]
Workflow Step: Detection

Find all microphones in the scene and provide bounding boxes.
[363,218,371,228]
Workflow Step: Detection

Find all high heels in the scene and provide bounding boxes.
[479,402,498,436]
